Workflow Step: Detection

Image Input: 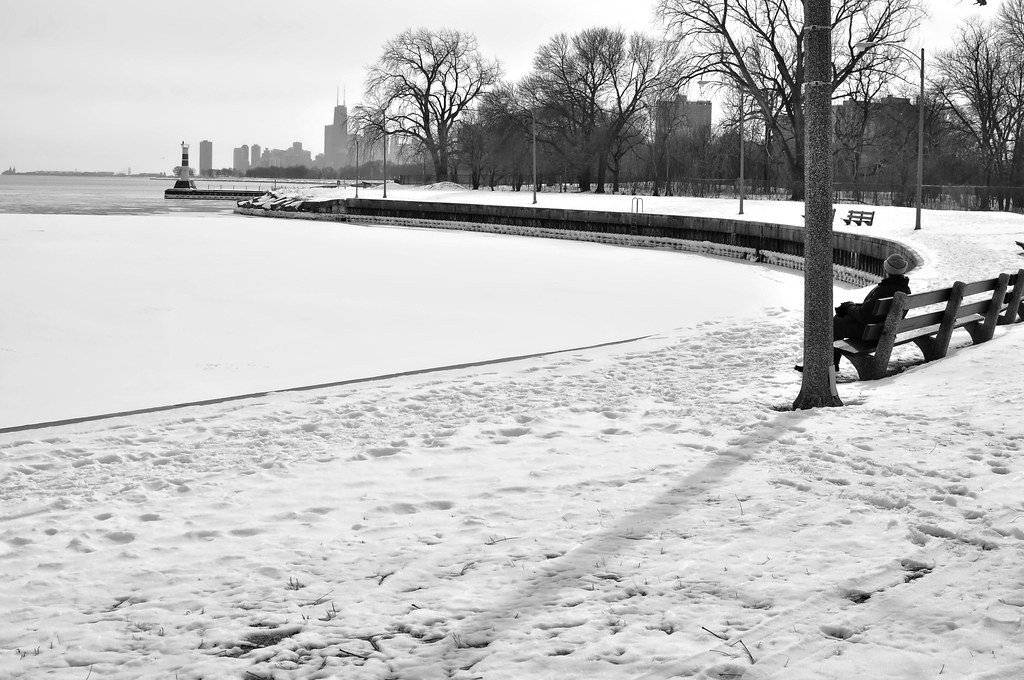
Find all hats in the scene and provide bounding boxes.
[884,254,909,275]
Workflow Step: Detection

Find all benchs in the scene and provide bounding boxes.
[841,210,874,226]
[834,269,1024,380]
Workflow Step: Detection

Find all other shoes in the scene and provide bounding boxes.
[794,365,803,372]
[835,367,839,371]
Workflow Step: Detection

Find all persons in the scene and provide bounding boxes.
[794,254,911,373]
